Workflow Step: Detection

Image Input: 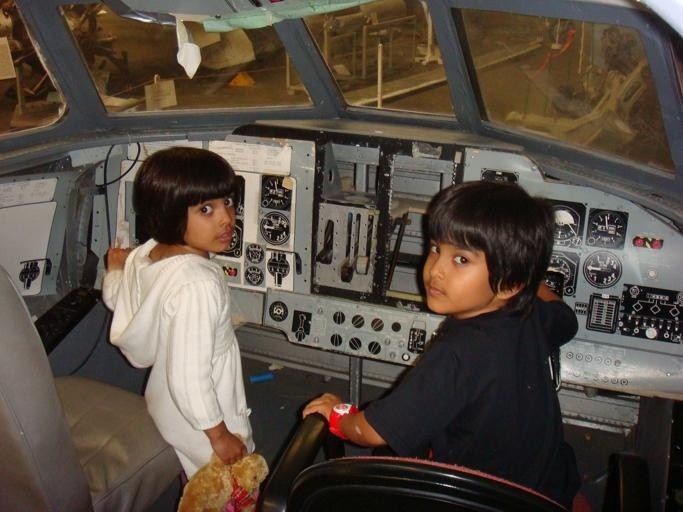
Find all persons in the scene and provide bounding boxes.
[302,181,581,512]
[102,147,256,479]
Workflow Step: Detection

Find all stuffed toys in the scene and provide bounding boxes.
[176,432,269,512]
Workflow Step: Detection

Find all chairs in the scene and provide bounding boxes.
[0,264,188,512]
[253,394,683,512]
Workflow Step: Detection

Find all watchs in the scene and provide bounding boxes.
[329,403,359,440]
